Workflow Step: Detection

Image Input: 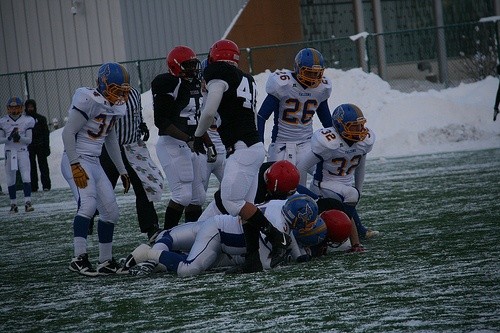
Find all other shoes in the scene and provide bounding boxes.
[147,229,162,242]
[88,219,94,234]
[31,185,50,191]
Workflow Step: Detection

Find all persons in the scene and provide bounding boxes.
[121,157,357,281]
[191,39,295,276]
[188,56,230,209]
[61,60,130,277]
[22,98,51,191]
[256,45,380,240]
[0,98,35,213]
[91,83,170,245]
[150,46,208,231]
[295,103,375,256]
[492,81,500,124]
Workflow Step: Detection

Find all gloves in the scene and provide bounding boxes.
[10,128,19,142]
[71,163,89,188]
[121,174,130,193]
[185,135,217,165]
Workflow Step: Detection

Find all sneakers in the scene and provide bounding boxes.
[359,227,379,238]
[69,244,167,276]
[9,204,19,213]
[24,201,34,212]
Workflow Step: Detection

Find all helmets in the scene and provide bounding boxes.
[294,48,325,78]
[7,96,24,111]
[198,59,208,80]
[331,103,365,141]
[96,63,131,96]
[167,46,196,76]
[208,40,241,67]
[263,160,352,248]
